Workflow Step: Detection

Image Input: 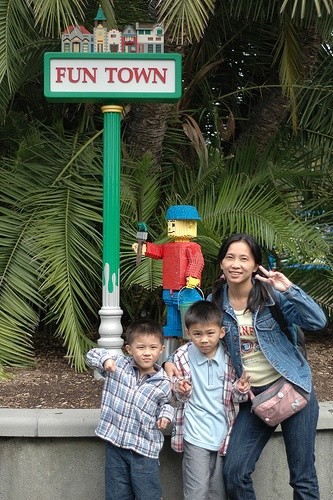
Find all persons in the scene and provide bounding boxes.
[83,322,181,499]
[164,299,253,500]
[191,230,333,500]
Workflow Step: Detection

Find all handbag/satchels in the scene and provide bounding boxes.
[250,377,311,427]
